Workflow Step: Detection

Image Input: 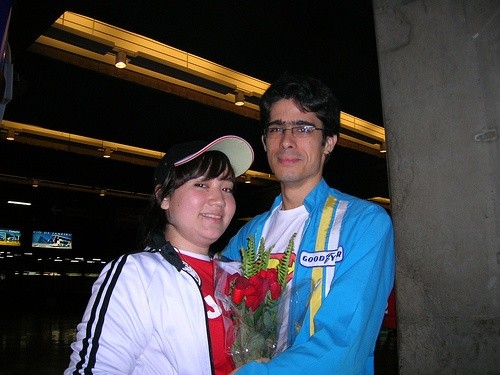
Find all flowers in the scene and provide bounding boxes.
[214,233,297,368]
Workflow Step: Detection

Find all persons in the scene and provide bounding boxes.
[64,135,255,375]
[221,76,396,375]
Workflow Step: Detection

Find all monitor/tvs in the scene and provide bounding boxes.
[31,231,72,249]
[0,229,22,247]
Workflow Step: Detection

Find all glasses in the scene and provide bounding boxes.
[264,125,324,138]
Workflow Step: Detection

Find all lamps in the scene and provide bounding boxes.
[99,189,105,196]
[103,147,111,158]
[32,179,38,188]
[380,141,387,153]
[115,50,126,69]
[245,175,252,183]
[5,128,15,141]
[234,90,246,107]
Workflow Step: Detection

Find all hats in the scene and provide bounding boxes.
[154,136,255,186]
